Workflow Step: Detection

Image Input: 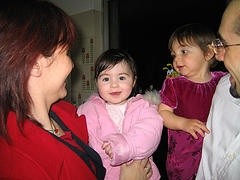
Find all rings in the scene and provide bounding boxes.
[145,167,149,173]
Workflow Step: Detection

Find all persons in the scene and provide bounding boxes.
[195,0,240,180]
[158,23,231,180]
[79,50,164,180]
[0,0,153,180]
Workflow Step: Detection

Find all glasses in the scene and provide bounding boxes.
[212,39,240,55]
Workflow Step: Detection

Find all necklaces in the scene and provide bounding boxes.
[46,120,61,137]
